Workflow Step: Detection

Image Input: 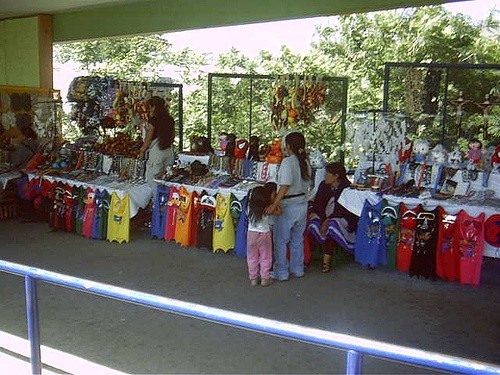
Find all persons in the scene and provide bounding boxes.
[262,132,312,281]
[303,161,357,274]
[247,186,284,287]
[138,95,175,228]
[12,126,46,222]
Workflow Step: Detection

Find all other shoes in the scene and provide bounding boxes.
[261,278,274,286]
[251,278,259,286]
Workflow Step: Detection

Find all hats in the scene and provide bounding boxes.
[177,160,208,176]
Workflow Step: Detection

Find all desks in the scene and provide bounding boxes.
[338,187,500,259]
[0,165,23,219]
[29,167,153,232]
[152,169,281,215]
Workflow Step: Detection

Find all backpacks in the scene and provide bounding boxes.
[21,143,46,171]
[153,116,172,150]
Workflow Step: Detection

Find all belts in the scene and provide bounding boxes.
[281,193,305,199]
